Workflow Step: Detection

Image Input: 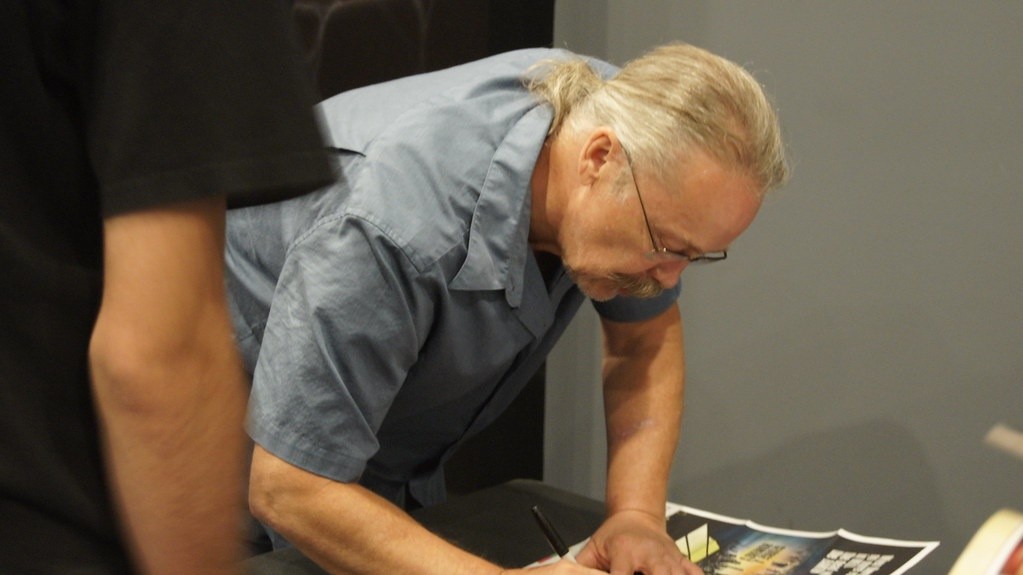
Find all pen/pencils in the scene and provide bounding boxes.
[532,502,576,563]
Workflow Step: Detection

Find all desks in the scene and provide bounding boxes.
[241,477,606,575]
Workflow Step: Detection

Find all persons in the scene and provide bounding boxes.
[221,46,784,575]
[0,0,334,575]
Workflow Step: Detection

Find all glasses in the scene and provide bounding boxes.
[617,144,728,265]
